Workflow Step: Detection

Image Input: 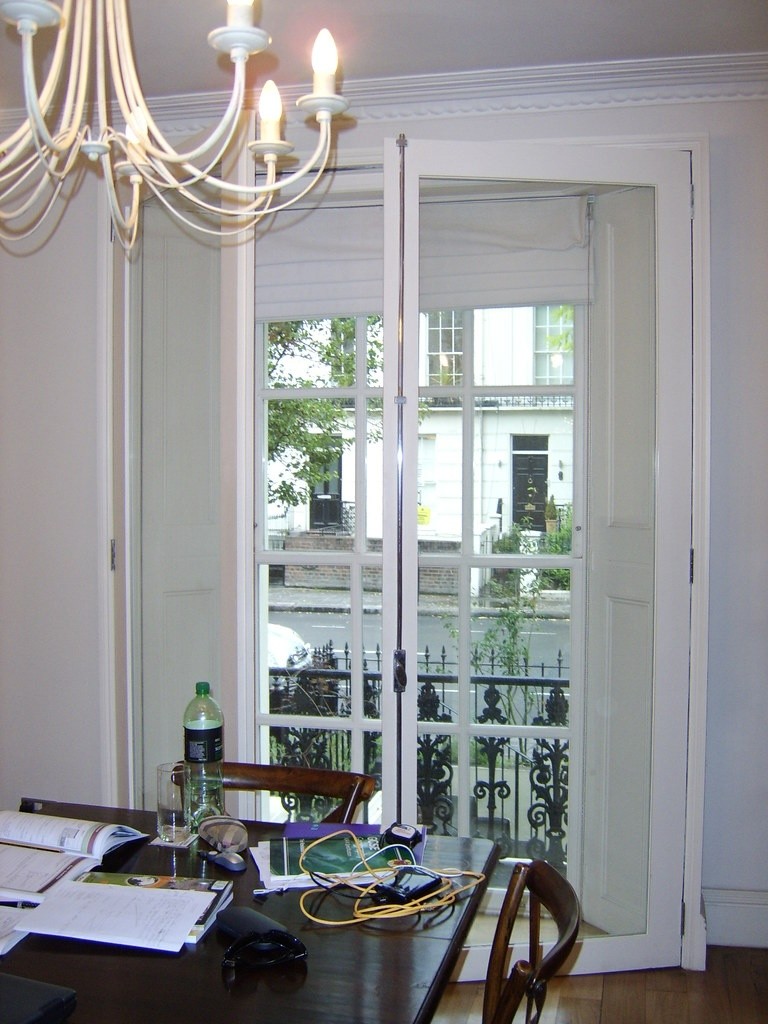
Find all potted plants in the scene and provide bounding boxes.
[544,495,557,534]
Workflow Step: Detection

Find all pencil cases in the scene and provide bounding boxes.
[216,905,288,942]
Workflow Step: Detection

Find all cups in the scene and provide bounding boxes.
[156,763,191,842]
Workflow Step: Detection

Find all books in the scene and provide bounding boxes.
[0,811,142,903]
[73,871,233,944]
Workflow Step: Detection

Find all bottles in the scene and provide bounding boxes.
[183,683,227,835]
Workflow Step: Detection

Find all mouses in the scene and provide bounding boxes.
[215,852,246,870]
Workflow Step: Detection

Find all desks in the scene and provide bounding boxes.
[0,802,500,1024]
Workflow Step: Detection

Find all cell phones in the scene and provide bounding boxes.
[375,868,442,904]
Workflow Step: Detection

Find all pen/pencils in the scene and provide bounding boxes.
[0,901,39,909]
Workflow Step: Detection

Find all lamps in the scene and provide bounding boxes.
[0,0,350,249]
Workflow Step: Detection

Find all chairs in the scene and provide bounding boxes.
[482,860,580,1024]
[173,761,374,825]
